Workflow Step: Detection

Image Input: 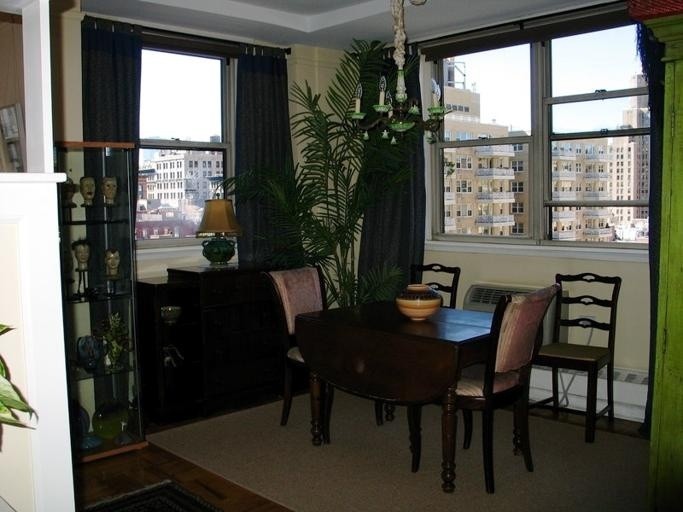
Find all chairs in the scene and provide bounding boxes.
[384,264,461,425]
[259,264,389,445]
[530,270,618,445]
[399,283,562,492]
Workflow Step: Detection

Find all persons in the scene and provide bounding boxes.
[79,176,96,207]
[70,239,91,271]
[60,177,77,209]
[99,177,117,205]
[101,249,121,276]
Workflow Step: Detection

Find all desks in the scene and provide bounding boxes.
[291,301,529,474]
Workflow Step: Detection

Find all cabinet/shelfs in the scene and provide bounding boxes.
[54,141,149,469]
[138,261,309,434]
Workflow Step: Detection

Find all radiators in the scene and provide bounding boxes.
[460,281,572,350]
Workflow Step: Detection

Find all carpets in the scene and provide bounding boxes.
[145,386,650,512]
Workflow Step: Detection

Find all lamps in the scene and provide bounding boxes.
[351,1,447,146]
[195,193,243,266]
